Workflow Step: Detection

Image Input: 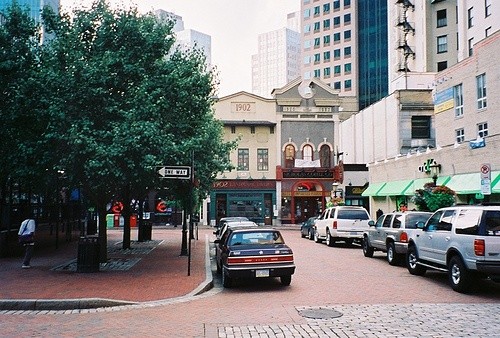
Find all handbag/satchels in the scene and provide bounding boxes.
[19,234,33,244]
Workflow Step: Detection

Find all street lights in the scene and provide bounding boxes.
[332,180,339,198]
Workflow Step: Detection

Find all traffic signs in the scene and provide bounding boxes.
[152,165,191,180]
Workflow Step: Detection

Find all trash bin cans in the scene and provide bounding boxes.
[77,236,100,273]
[106,213,137,228]
[138,222,152,241]
[87,220,97,235]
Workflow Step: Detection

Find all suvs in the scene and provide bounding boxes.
[363,212,434,266]
[314,205,371,247]
[407,201,500,293]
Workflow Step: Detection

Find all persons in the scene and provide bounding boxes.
[18,212,35,269]
[376,209,383,219]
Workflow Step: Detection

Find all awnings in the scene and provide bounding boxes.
[492,179,500,193]
[378,180,414,196]
[361,182,386,196]
[404,176,450,196]
[445,172,500,194]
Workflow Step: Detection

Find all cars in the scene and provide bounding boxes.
[214,226,296,288]
[213,217,264,244]
[300,217,318,240]
